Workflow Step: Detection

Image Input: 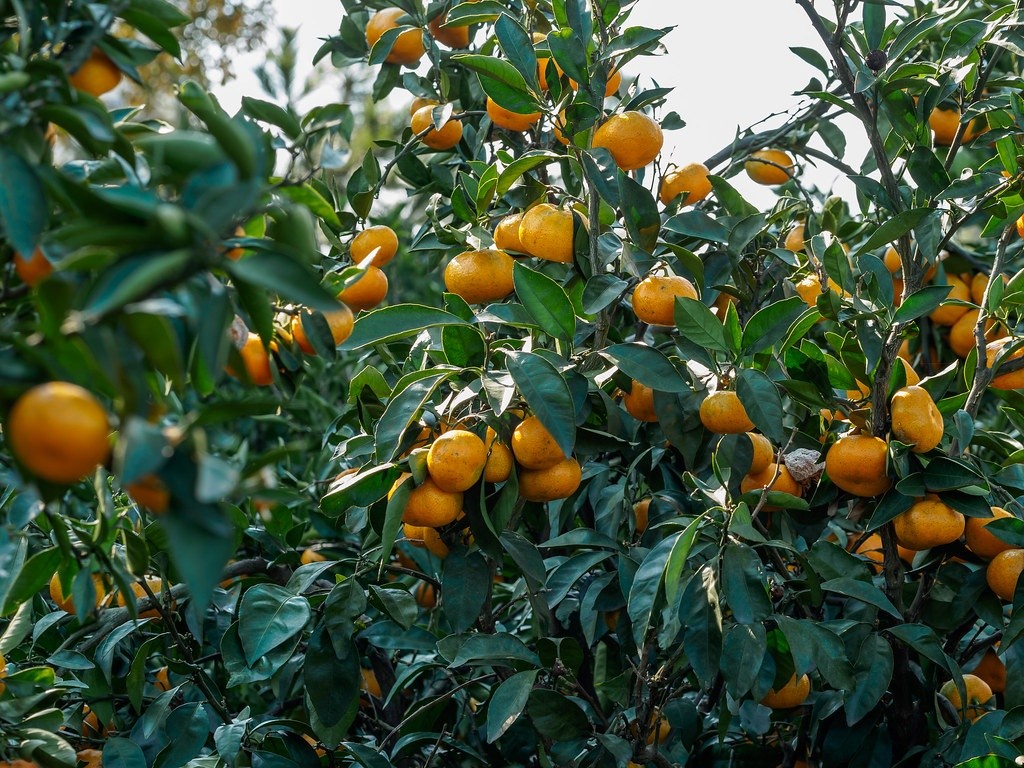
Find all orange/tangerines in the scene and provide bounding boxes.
[0,5,1024,768]
